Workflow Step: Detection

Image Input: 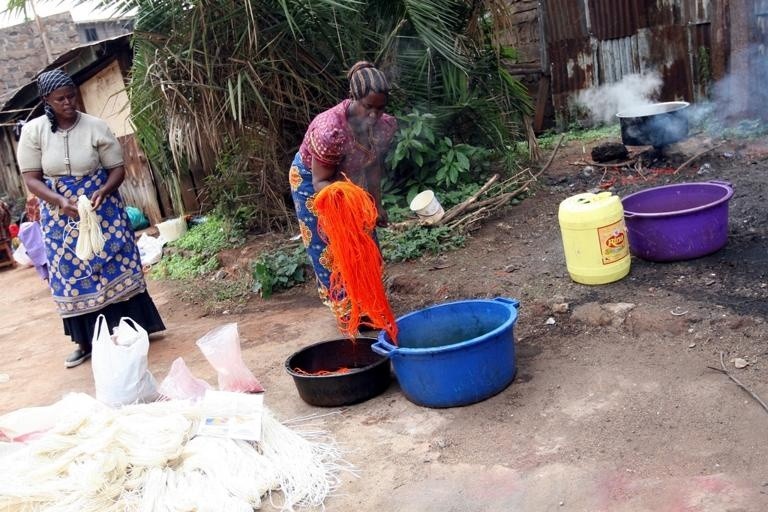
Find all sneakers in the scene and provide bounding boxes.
[64,347,91,369]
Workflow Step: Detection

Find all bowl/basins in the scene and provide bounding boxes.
[620,179,735,265]
[283,335,392,408]
[370,296,522,410]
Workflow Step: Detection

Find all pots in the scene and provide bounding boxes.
[616,101,692,147]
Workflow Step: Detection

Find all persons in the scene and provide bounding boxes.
[288,60,400,331]
[16,69,167,368]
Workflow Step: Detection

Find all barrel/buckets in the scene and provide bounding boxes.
[559,193,632,283]
[410,189,444,226]
